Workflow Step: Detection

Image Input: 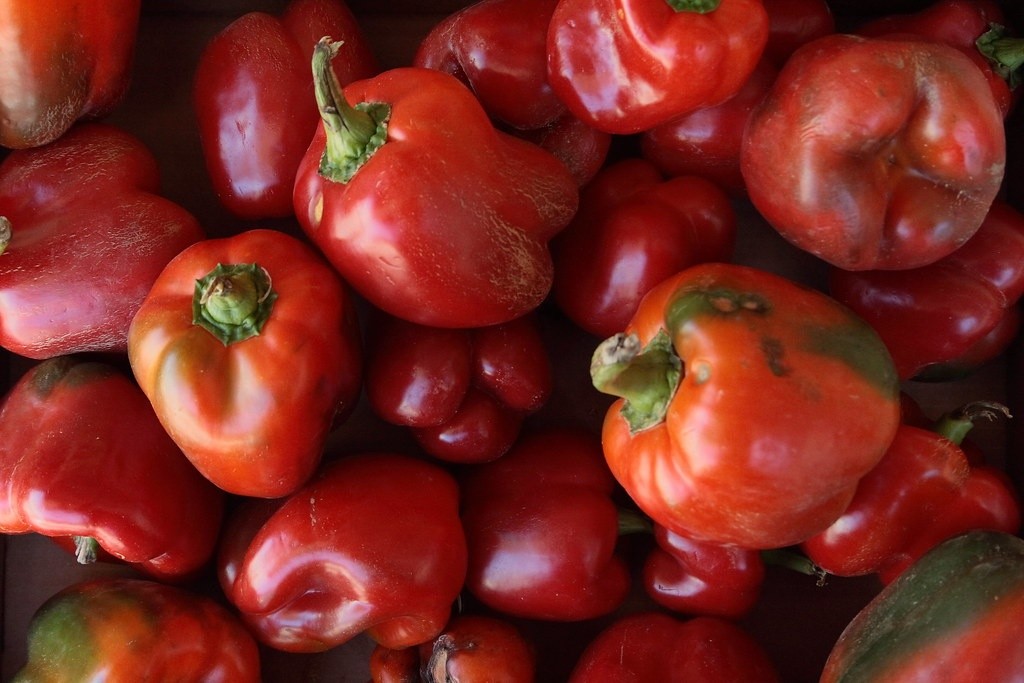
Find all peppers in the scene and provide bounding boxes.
[0,0,1024,683]
